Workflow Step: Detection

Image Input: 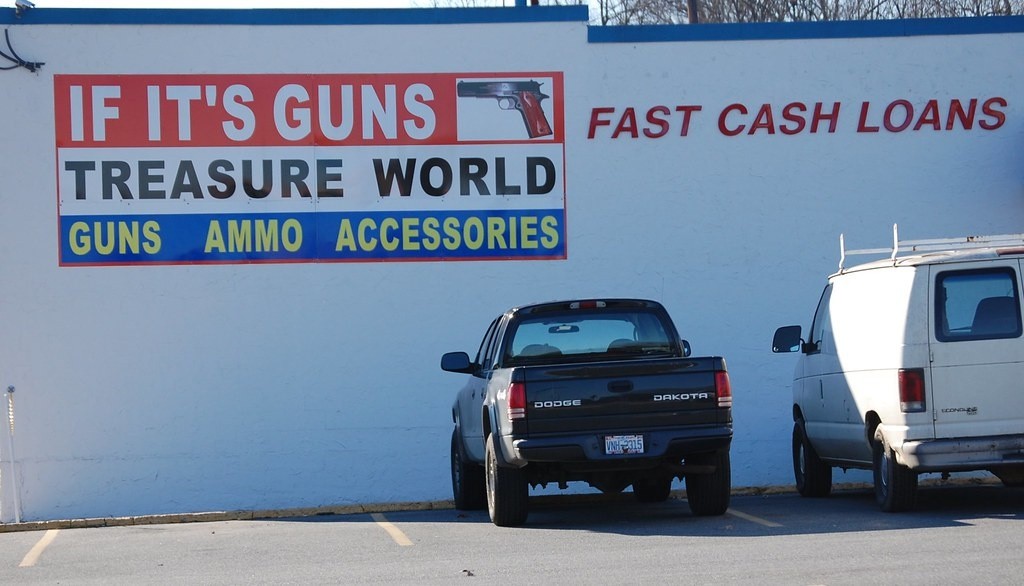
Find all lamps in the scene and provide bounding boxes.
[15,0,35,19]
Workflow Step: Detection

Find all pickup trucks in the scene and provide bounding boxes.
[442,300,732,529]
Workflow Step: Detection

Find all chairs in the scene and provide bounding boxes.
[607,339,637,352]
[971,296,1018,335]
[521,344,562,356]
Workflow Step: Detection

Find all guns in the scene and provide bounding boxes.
[456,79,554,139]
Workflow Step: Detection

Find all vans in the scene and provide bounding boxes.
[771,222,1024,512]
